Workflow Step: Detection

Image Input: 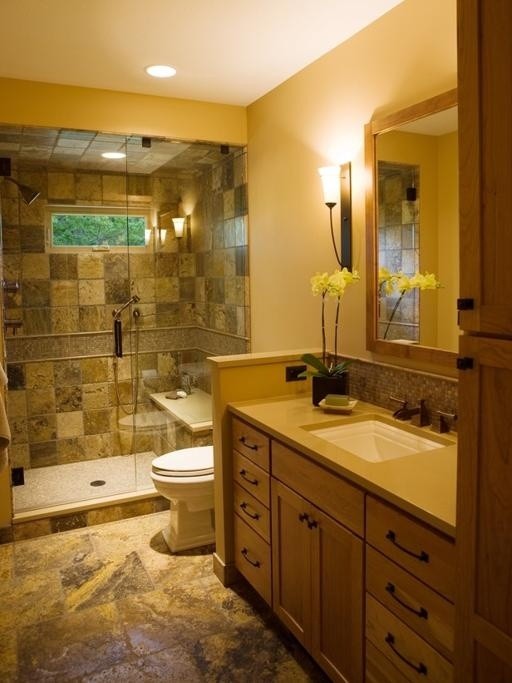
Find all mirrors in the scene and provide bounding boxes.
[364,84,459,369]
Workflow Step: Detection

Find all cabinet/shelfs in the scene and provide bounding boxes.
[365,485,459,682]
[228,413,271,609]
[270,436,364,683]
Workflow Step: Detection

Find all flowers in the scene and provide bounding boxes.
[375,265,441,339]
[298,267,359,377]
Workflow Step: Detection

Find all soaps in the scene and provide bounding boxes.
[325,393,349,405]
[177,390,187,399]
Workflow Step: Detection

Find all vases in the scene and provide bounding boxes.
[312,370,349,405]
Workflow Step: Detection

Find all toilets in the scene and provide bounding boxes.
[118,410,178,454]
[150,445,216,554]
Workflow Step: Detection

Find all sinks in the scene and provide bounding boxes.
[297,411,458,465]
[149,388,213,432]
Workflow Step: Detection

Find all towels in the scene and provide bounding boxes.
[1,363,12,454]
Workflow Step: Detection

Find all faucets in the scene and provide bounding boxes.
[387,398,427,421]
[180,375,193,395]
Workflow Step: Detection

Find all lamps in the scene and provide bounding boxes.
[2,171,41,205]
[317,162,351,273]
[142,135,153,151]
[171,214,186,246]
[220,143,229,154]
